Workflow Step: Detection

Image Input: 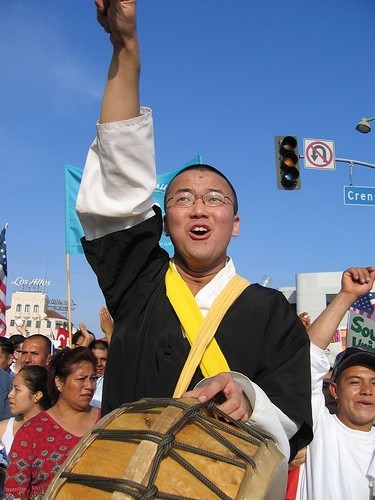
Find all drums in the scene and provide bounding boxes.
[41,395,288,500]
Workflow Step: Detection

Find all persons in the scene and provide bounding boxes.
[0,265,375,500]
[73,0,314,500]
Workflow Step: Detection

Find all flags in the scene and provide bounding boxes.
[347,291,375,353]
[0,228,7,337]
[65,154,203,256]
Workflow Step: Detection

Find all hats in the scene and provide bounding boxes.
[330,347,375,382]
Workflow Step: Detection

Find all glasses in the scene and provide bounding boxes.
[165,189,236,216]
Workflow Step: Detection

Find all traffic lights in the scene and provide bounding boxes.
[274,135,302,191]
